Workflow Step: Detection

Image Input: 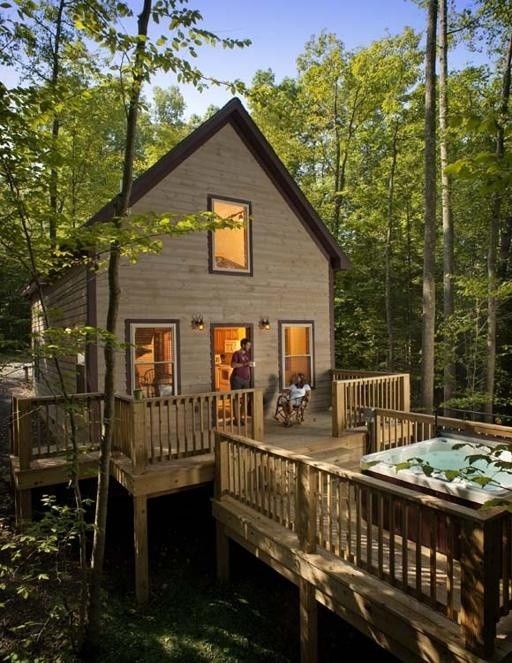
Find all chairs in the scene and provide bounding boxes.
[141,369,172,397]
[272,389,311,427]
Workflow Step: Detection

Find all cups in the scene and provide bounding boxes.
[134,389,143,400]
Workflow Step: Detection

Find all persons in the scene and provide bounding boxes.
[227,337,255,426]
[279,371,312,427]
